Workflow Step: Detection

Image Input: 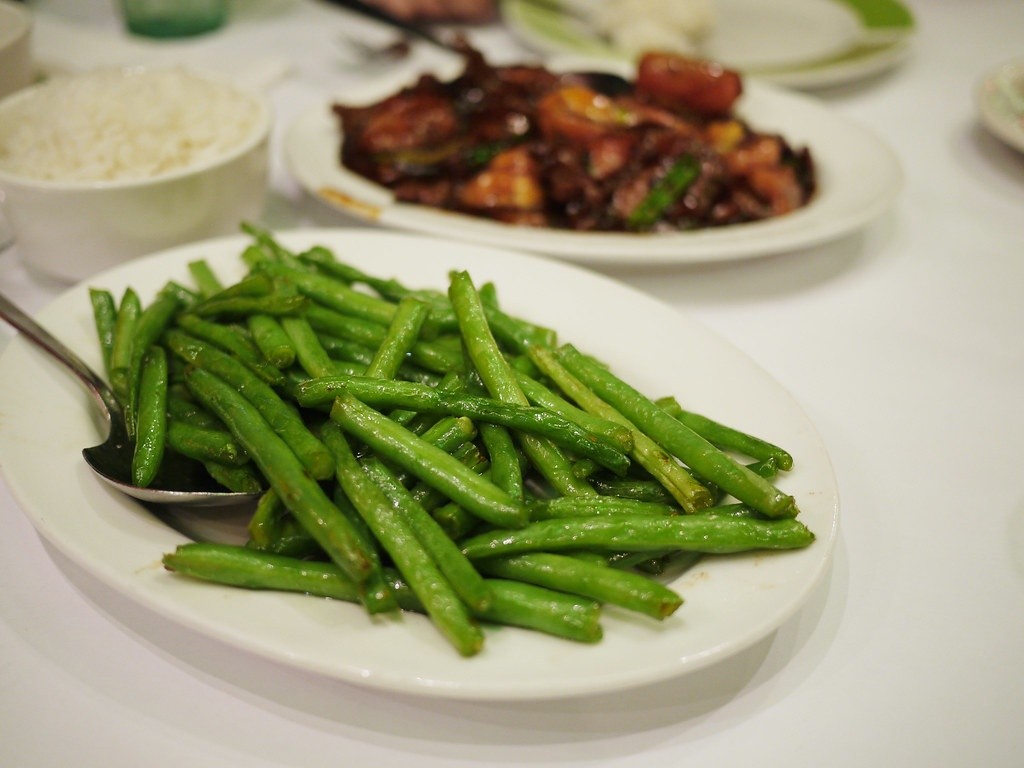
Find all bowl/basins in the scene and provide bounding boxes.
[0,63,272,280]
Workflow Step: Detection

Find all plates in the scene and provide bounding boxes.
[504,0,917,80]
[0,229,839,699]
[979,57,1024,152]
[287,53,905,259]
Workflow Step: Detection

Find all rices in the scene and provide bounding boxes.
[0,61,266,181]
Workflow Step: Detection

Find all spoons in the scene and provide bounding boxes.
[0,293,252,504]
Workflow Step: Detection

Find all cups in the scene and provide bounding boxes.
[116,0,230,38]
[0,0,31,96]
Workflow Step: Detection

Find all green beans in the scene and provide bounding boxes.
[87,222,815,657]
